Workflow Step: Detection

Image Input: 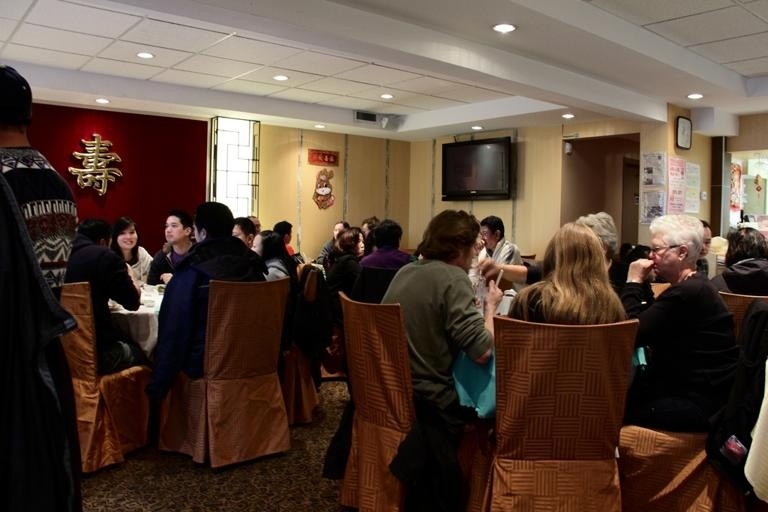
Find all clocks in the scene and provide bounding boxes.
[675,115,692,150]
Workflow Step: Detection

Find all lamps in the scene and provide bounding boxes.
[565,142,573,156]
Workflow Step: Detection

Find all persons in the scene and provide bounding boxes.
[315,214,523,307]
[382,207,505,436]
[61,204,308,427]
[0,67,78,302]
[478,210,767,472]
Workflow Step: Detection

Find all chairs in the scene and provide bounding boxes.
[718,290,768,346]
[152,275,292,469]
[337,290,416,512]
[650,282,672,300]
[458,315,641,512]
[278,263,336,423]
[619,423,745,512]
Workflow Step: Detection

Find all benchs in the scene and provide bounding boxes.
[57,281,152,473]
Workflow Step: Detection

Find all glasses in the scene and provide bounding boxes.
[648,245,681,253]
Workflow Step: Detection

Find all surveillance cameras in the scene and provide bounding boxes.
[565,141,574,155]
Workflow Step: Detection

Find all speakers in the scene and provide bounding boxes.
[381,117,400,130]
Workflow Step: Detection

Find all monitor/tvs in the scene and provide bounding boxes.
[441,136,511,195]
